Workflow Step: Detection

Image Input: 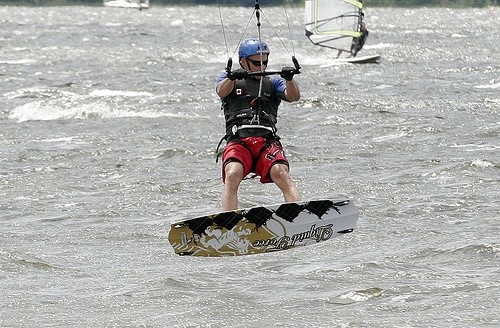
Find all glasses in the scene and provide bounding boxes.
[246,57,268,66]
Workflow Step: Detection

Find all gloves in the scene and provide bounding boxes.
[231,69,248,80]
[281,67,296,81]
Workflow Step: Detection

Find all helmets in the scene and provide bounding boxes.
[238,38,270,59]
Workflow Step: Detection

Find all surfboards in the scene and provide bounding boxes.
[167,198,360,257]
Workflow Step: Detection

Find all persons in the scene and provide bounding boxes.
[330,20,370,60]
[214,37,302,213]
